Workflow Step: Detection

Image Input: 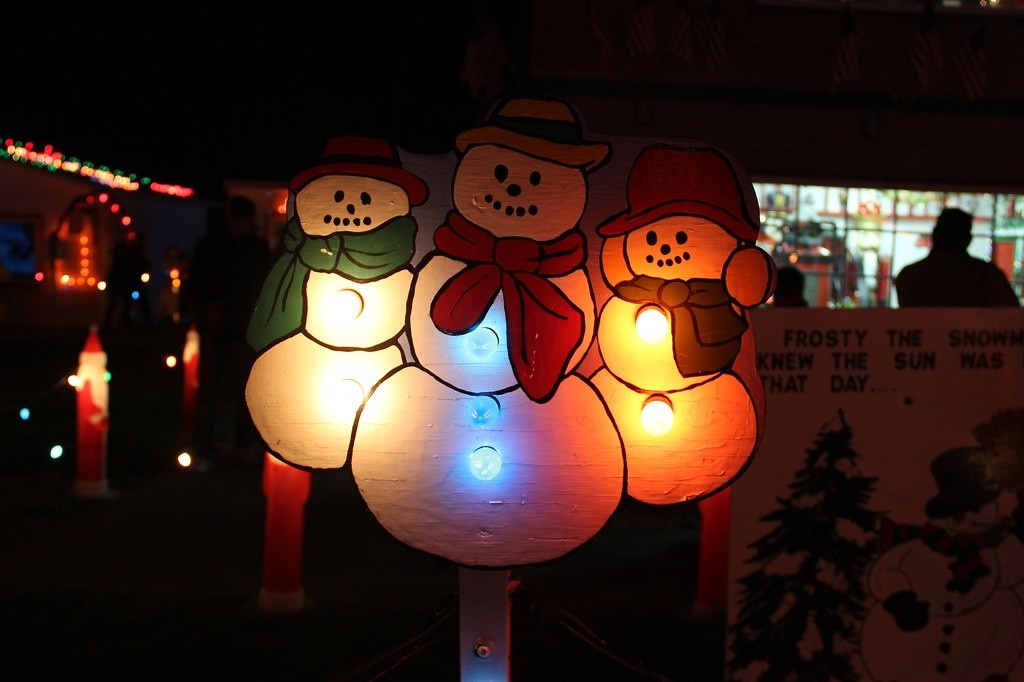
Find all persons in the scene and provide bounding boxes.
[98,241,193,489]
[773,266,807,307]
[179,194,274,474]
[896,208,1020,306]
